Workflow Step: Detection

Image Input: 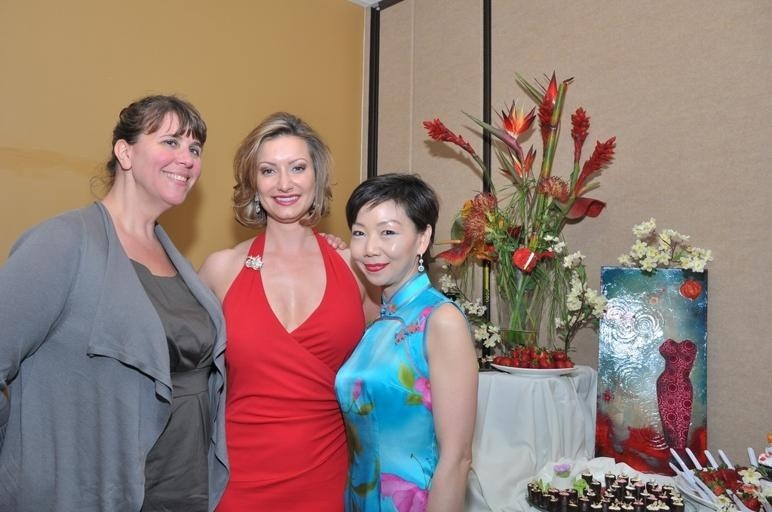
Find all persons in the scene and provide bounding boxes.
[333,169,481,511]
[200,110,388,510]
[2,94,350,512]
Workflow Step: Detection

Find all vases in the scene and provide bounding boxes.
[492,252,550,360]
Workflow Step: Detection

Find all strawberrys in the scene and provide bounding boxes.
[493,345,574,369]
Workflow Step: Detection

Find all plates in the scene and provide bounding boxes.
[672,467,723,511]
[489,361,578,377]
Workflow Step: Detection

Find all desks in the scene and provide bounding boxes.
[465,364,598,512]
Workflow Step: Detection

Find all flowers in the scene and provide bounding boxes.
[422,71,619,350]
